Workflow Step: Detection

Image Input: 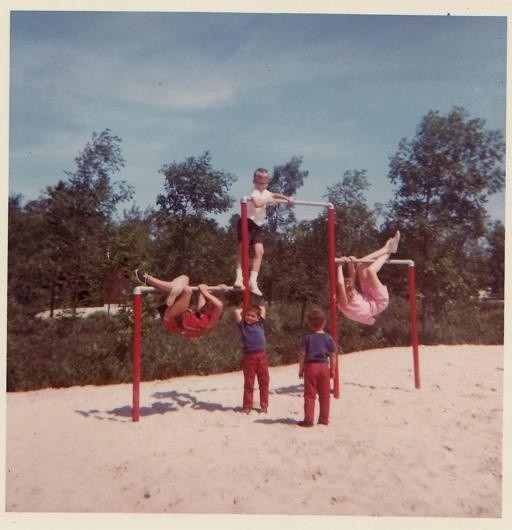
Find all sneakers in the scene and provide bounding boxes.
[135,269,149,286]
[386,231,400,255]
[249,281,262,297]
[233,277,243,289]
[260,408,267,417]
[241,408,250,417]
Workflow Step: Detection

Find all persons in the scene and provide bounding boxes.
[235,305,269,416]
[297,308,337,427]
[136,268,228,339]
[233,167,294,297]
[335,230,401,325]
